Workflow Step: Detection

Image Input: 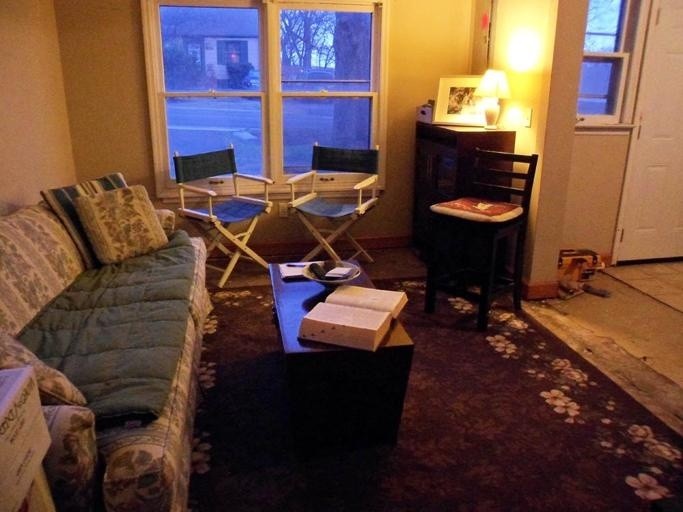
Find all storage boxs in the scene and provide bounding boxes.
[0,365,52,511]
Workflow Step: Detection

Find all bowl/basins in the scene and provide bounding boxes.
[301,258,360,287]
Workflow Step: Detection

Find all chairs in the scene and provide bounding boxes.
[172,141,381,290]
[423,147,538,331]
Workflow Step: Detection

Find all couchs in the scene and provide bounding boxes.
[0,200,213,512]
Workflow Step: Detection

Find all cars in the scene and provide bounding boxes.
[240,66,334,104]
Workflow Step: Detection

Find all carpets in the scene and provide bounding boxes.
[185,276,683,512]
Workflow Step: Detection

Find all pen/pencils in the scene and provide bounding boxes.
[287,264,306,267]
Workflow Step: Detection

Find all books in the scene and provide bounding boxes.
[278,261,410,352]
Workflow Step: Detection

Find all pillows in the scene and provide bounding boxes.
[0,328,88,407]
[39,172,168,270]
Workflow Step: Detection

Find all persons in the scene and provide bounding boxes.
[226,51,254,89]
[448,86,473,114]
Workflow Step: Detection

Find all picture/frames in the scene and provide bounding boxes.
[430,75,499,127]
